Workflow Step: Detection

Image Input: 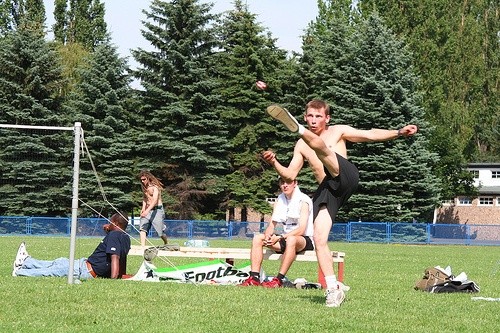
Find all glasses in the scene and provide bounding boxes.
[278,179,294,185]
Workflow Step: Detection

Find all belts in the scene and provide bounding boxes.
[151,206,162,210]
[86,260,97,278]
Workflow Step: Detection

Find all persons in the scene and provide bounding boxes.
[237,99,418,308]
[12,212,130,281]
[138,170,168,246]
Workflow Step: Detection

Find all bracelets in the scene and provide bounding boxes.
[398,129,401,136]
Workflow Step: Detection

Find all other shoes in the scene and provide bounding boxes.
[262,277,282,288]
[237,276,260,286]
[12,260,22,277]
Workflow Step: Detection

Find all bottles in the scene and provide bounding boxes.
[184,240,209,248]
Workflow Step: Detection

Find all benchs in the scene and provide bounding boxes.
[127,242,346,289]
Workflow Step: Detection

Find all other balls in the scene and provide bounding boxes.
[255,81,268,91]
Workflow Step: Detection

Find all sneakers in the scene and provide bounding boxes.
[324,281,346,307]
[15,241,30,267]
[267,105,299,133]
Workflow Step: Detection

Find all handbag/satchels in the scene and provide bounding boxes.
[414,266,452,291]
